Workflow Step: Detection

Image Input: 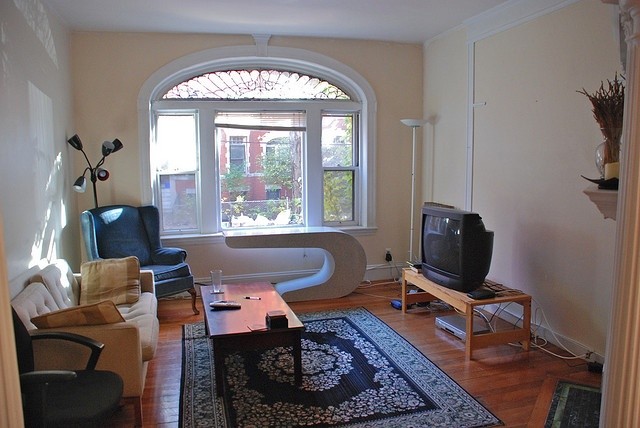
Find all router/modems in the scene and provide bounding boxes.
[390,298,413,309]
[467,289,495,300]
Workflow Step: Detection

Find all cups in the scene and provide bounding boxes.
[210,269,222,293]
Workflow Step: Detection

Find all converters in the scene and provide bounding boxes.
[587,362,602,374]
[386,254,392,262]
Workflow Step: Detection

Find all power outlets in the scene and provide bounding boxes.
[385,246,391,258]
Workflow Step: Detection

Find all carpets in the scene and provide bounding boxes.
[542,378,604,428]
[178,304,504,427]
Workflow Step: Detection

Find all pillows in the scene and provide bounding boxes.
[30,300,127,329]
[78,255,141,307]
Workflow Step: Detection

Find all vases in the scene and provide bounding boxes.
[593,128,627,180]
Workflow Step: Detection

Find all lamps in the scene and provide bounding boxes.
[67,133,92,168]
[73,166,92,193]
[92,167,110,181]
[94,138,124,173]
[400,116,430,264]
[94,141,114,174]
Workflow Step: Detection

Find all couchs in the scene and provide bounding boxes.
[8,255,161,427]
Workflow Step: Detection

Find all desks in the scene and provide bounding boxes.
[401,266,532,361]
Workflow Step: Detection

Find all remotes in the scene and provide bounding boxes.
[210,301,241,308]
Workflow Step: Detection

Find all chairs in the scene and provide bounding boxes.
[81,204,200,315]
[11,301,124,428]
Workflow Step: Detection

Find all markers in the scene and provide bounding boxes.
[246,297,261,300]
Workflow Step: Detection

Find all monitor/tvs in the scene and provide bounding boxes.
[420,202,494,292]
